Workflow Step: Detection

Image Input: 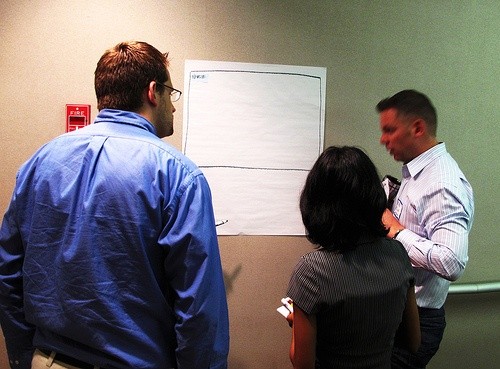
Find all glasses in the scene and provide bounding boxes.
[153,78,182,102]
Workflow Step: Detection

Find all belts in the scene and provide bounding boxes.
[37,348,102,369]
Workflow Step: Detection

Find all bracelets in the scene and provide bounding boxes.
[392,229,402,240]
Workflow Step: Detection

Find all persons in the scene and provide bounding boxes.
[286,146,420,369]
[367,90,476,369]
[0,41,230,368]
